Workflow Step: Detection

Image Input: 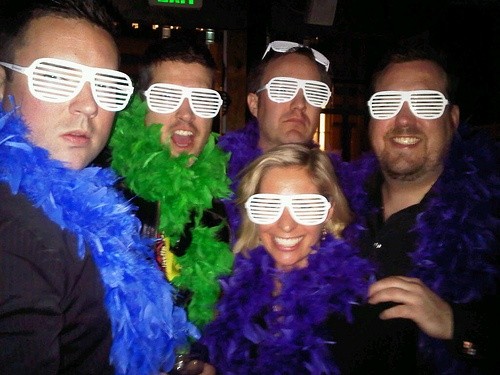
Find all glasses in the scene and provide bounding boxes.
[0,57,134,112]
[244,194,331,226]
[256,76,332,109]
[140,82,223,119]
[367,90,448,120]
[262,41,330,72]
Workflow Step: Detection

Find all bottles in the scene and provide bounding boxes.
[205,29,217,65]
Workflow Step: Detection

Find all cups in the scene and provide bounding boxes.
[175,345,204,375]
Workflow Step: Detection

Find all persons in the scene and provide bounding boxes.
[216,41,342,244]
[185,143,419,375]
[335,55,500,375]
[107,40,235,375]
[1,0,203,375]
